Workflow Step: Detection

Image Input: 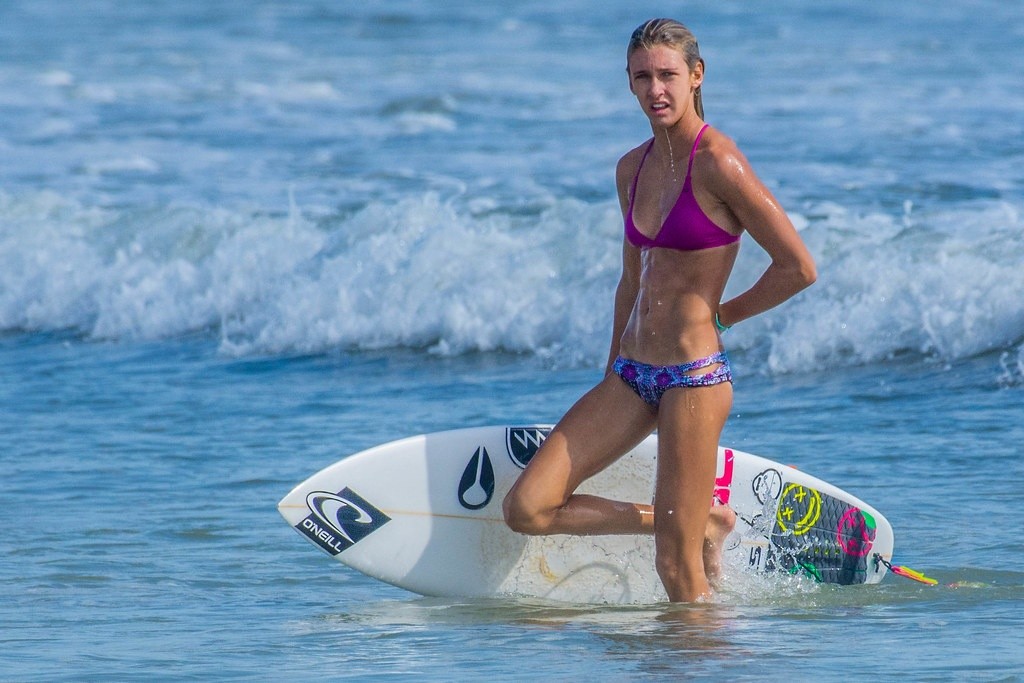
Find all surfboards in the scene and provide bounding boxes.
[277,422,895,606]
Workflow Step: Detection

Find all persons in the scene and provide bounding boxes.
[501,18,818,603]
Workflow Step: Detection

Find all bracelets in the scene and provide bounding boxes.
[715,313,732,331]
